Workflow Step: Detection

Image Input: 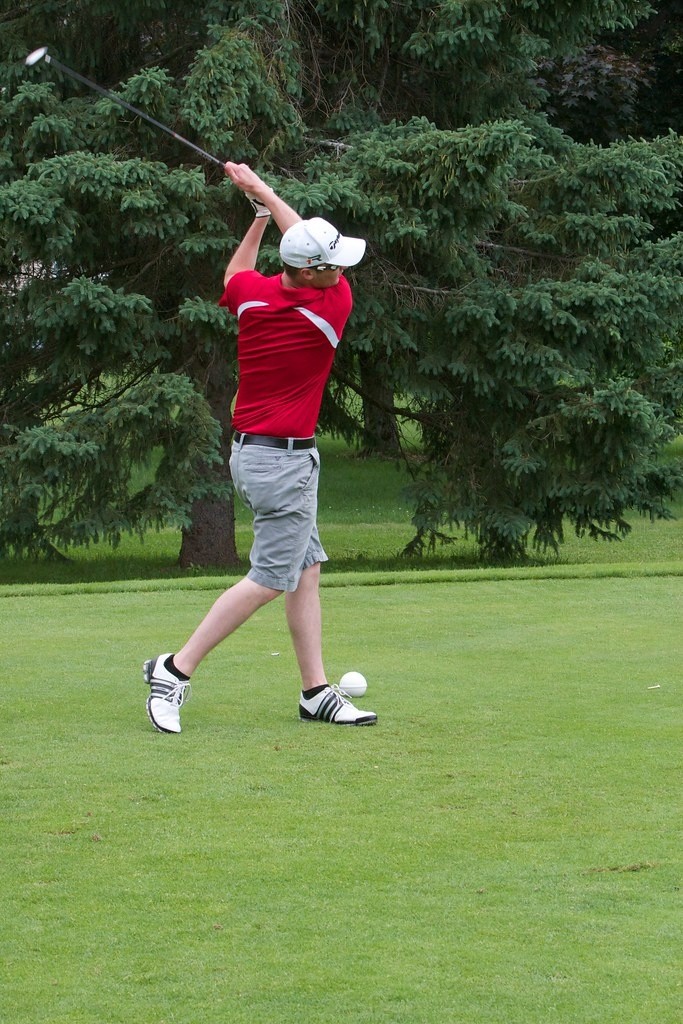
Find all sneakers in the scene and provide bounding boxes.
[299,684,378,725]
[143,652,192,733]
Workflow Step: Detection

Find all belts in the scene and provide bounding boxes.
[233,431,315,450]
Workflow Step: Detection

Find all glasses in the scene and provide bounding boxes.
[306,265,339,271]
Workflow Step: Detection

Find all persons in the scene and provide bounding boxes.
[144,161,378,732]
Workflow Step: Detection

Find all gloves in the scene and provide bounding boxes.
[245,181,273,218]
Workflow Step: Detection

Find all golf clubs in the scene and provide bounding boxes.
[25,46,226,169]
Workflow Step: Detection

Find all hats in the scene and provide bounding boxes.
[280,217,366,269]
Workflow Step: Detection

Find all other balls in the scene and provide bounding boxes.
[340,672,368,698]
